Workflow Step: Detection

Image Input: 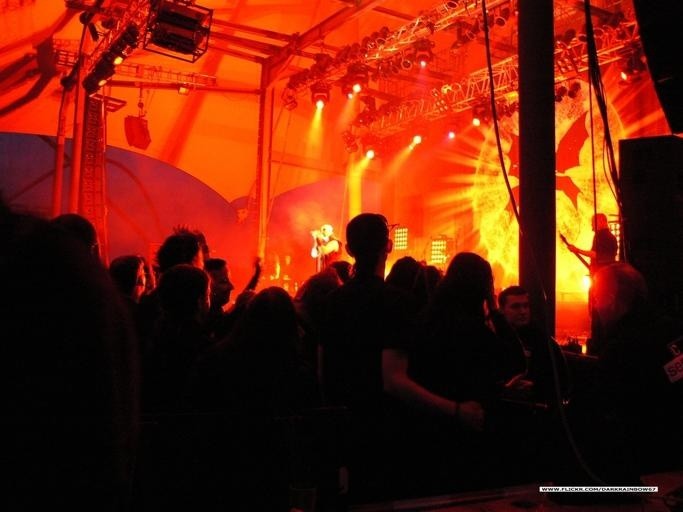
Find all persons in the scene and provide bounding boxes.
[0,194,683,509]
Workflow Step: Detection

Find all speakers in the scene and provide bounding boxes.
[619,135,683,474]
[633,0,683,134]
